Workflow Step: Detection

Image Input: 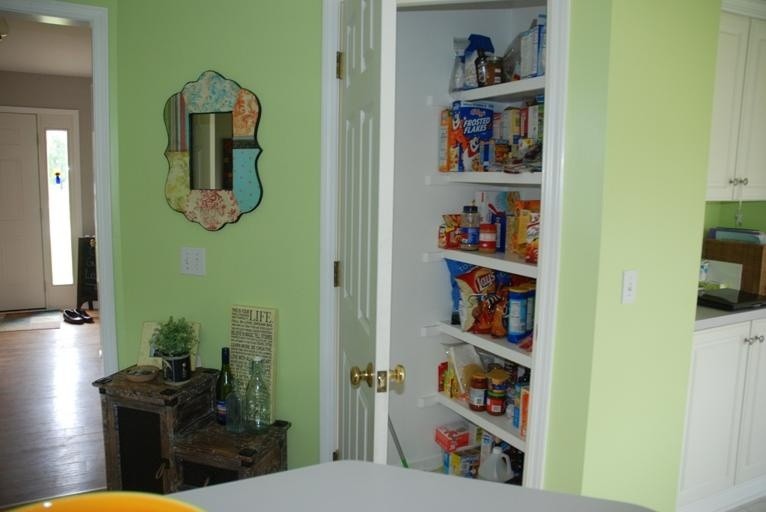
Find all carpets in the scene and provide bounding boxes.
[1,310,62,331]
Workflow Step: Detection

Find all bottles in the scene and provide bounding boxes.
[473,56,502,86]
[457,205,498,253]
[467,371,507,417]
[245,357,270,433]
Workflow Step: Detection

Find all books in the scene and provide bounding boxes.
[228,304,279,427]
[137,320,200,372]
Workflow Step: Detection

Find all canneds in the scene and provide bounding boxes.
[487,390,507,416]
[468,372,488,411]
[479,224,497,254]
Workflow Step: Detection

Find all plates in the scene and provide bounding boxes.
[125,365,159,381]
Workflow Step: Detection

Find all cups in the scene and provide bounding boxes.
[225,391,249,433]
[216,347,238,426]
[699,260,709,282]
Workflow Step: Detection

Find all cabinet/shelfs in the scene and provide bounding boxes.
[90,359,294,495]
[413,3,561,491]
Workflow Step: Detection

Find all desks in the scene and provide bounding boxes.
[6,460,654,512]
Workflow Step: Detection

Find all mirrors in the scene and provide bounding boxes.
[160,70,265,233]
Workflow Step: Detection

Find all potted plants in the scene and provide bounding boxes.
[147,316,200,386]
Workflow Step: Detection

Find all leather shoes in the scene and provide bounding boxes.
[75,309,93,323]
[64,310,83,324]
